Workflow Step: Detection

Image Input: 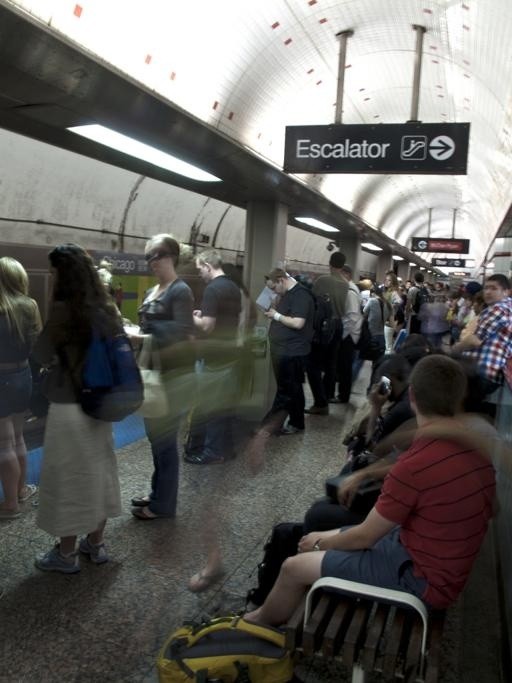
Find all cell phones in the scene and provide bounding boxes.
[377,375,391,396]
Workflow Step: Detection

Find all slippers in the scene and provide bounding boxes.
[1,505,22,522]
[187,567,229,593]
[18,485,36,503]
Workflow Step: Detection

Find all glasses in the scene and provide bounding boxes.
[145,251,173,260]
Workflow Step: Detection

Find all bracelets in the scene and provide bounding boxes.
[273,312,282,320]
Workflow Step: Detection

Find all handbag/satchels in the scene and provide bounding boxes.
[136,333,169,420]
[156,615,295,683]
[252,564,274,607]
[269,524,303,584]
[364,297,385,355]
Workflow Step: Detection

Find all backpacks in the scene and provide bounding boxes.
[54,297,145,422]
[413,285,429,315]
[246,521,308,609]
[287,287,344,350]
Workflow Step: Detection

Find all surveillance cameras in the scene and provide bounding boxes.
[326,245,333,251]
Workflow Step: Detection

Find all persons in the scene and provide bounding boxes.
[305,253,348,414]
[429,274,512,401]
[131,234,195,522]
[37,242,123,572]
[234,357,498,632]
[341,356,417,449]
[189,250,240,463]
[303,363,511,530]
[330,264,360,402]
[353,267,410,332]
[409,271,430,326]
[1,258,42,520]
[263,270,310,436]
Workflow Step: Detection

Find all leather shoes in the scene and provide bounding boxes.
[304,409,310,413]
[328,396,349,404]
[131,496,157,508]
[184,454,222,466]
[309,406,329,415]
[132,506,171,521]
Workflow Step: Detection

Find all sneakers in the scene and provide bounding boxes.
[249,429,272,440]
[34,544,84,575]
[77,537,110,566]
[281,424,305,435]
[224,453,237,460]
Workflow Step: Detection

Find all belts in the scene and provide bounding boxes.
[2,361,31,372]
